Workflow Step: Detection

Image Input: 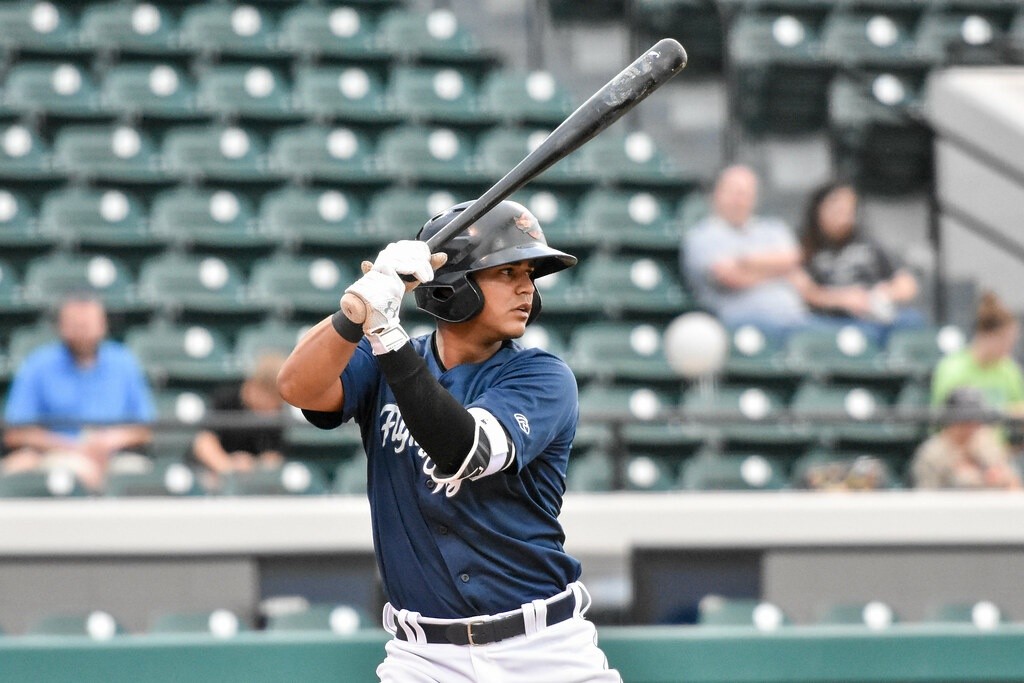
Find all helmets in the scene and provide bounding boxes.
[414,199,578,325]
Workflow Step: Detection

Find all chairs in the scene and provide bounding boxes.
[822,14,912,53]
[3,61,99,107]
[385,65,474,109]
[79,2,175,49]
[0,0,76,47]
[195,66,287,110]
[292,66,384,110]
[278,3,372,53]
[729,14,815,57]
[919,13,1000,54]
[101,63,191,107]
[829,74,916,131]
[477,68,570,112]
[177,3,275,49]
[0,128,1010,637]
[374,7,474,50]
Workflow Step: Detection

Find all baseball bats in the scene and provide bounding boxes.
[339,38,688,325]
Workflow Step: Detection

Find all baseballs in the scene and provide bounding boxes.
[663,311,728,378]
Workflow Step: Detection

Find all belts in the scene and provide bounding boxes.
[394,593,575,646]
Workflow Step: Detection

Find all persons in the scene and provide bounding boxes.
[905,389,1024,491]
[680,160,931,353]
[275,200,624,683]
[184,358,294,488]
[0,294,159,489]
[931,294,1024,440]
